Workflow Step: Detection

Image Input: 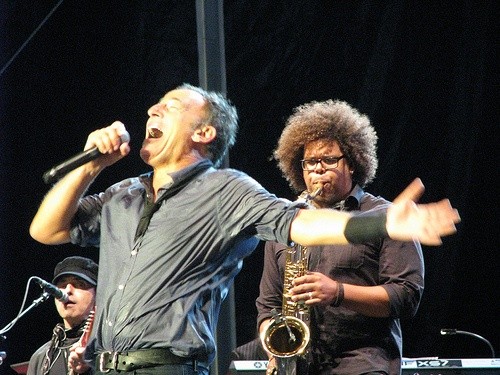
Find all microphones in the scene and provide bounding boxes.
[32,276,69,303]
[42,132,130,185]
[440,328,495,358]
[280,318,297,348]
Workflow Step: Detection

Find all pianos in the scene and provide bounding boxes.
[224,356,500,375]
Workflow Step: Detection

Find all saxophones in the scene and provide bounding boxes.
[260,181,325,375]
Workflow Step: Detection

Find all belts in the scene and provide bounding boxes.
[96,348,209,373]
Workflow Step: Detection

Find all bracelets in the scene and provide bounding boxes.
[343,206,392,246]
[329,282,344,307]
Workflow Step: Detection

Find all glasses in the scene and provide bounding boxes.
[299,155,347,170]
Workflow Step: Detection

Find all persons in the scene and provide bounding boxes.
[29,83,464,375]
[253,100,426,375]
[26,256,99,375]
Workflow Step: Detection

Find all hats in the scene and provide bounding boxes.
[52,256,98,286]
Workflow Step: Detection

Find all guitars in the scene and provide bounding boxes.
[66,305,97,375]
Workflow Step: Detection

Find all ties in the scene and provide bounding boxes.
[135,160,213,243]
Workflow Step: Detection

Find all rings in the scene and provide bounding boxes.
[72,360,77,365]
[308,292,313,299]
